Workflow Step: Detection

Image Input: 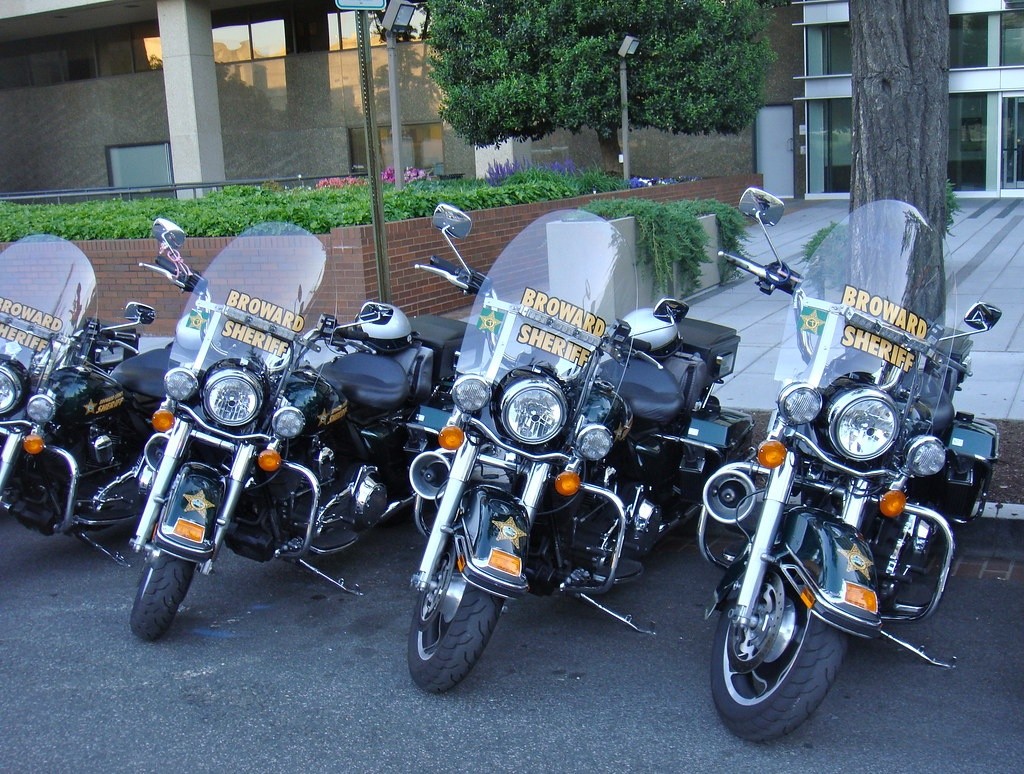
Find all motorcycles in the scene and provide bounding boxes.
[405,202,760,694]
[116,220,489,644]
[0,232,198,572]
[697,186,1003,744]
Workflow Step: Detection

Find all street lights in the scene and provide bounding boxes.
[379,3,418,195]
[616,35,641,183]
